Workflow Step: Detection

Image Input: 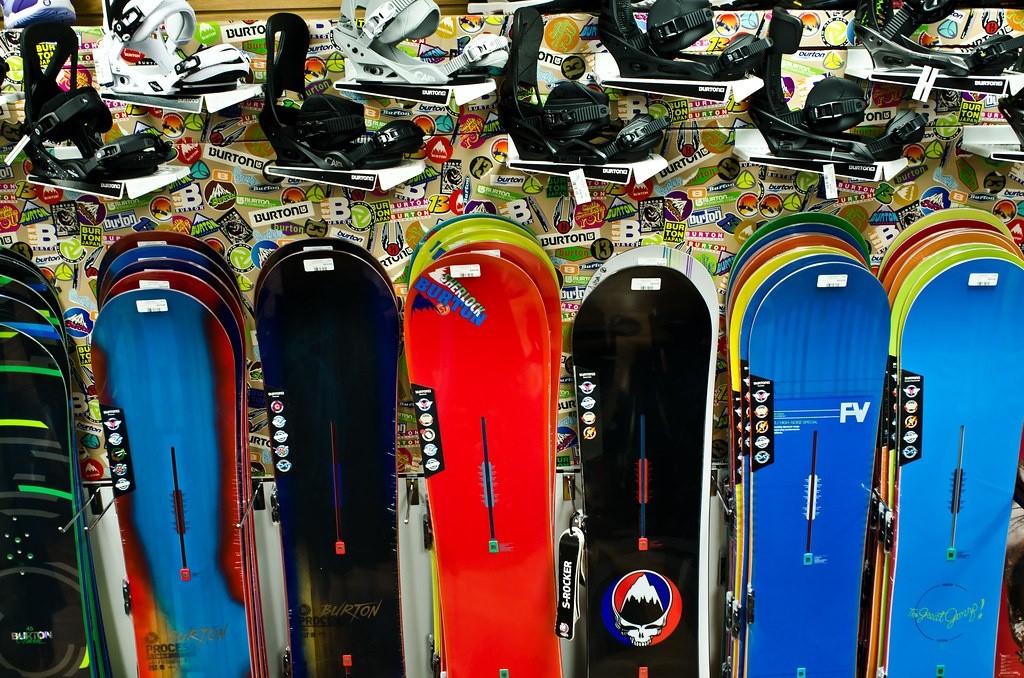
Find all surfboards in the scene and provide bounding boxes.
[0,200,1024,678]
